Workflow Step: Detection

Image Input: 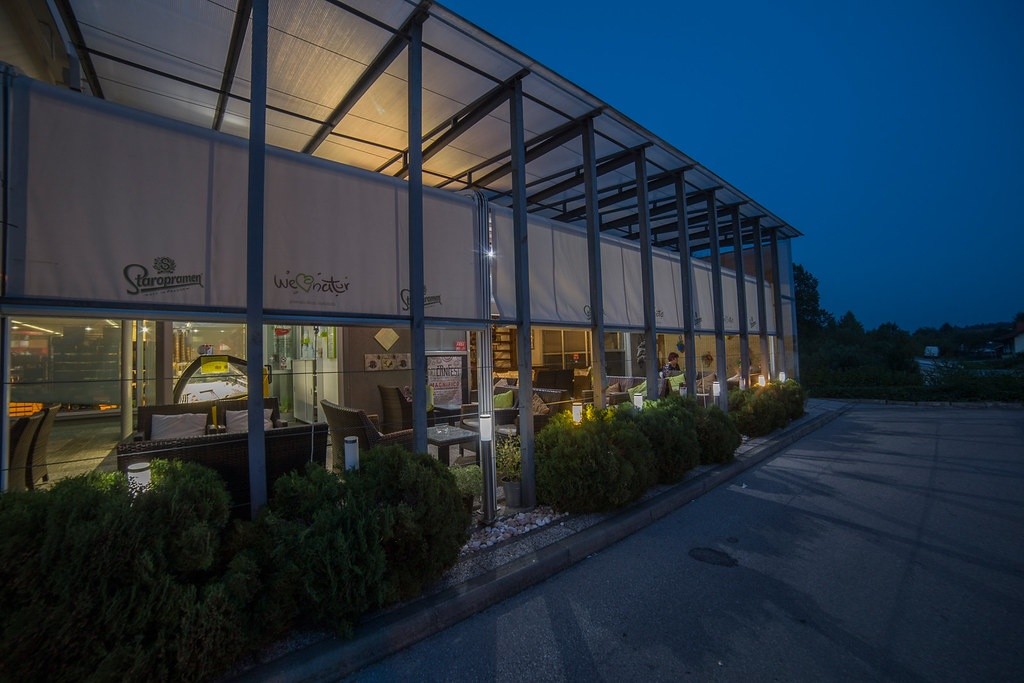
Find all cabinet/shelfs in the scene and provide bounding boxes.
[492,325,518,373]
[291,358,338,425]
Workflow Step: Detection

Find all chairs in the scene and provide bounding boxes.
[377,385,435,435]
[319,400,414,475]
[9,403,63,492]
[216,396,288,433]
[133,400,217,442]
[460,366,763,468]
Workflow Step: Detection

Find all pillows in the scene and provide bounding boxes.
[606,382,621,397]
[696,371,717,394]
[151,414,207,440]
[493,390,513,408]
[666,373,686,391]
[226,409,275,433]
[532,393,549,415]
[628,380,647,403]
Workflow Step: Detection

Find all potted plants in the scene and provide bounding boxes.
[494,423,521,507]
[447,465,485,513]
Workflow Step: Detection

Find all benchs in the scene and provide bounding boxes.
[118,422,329,506]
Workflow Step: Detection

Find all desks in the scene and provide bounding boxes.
[426,425,480,469]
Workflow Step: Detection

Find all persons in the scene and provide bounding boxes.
[697,354,717,406]
[738,356,761,374]
[566,353,582,399]
[663,352,682,398]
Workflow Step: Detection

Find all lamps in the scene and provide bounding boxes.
[344,436,359,469]
[680,387,687,400]
[572,403,583,423]
[738,378,745,389]
[479,415,491,442]
[758,375,765,386]
[779,372,785,382]
[633,393,642,411]
[127,463,152,501]
[712,382,720,396]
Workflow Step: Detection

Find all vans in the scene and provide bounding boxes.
[924,346,943,358]
